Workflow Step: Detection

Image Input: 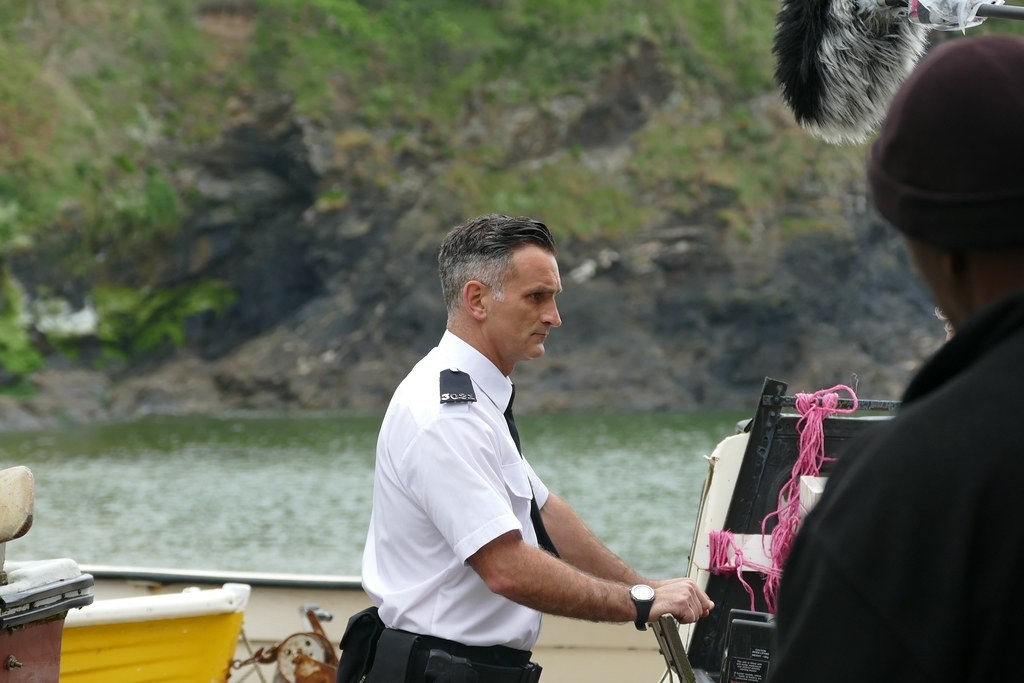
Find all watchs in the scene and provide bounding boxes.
[628,584,656,631]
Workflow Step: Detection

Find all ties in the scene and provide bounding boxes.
[504,384,560,560]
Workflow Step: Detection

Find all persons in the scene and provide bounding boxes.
[755,28,1024,683]
[357,216,718,683]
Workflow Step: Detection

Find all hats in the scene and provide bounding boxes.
[866,33,1024,251]
